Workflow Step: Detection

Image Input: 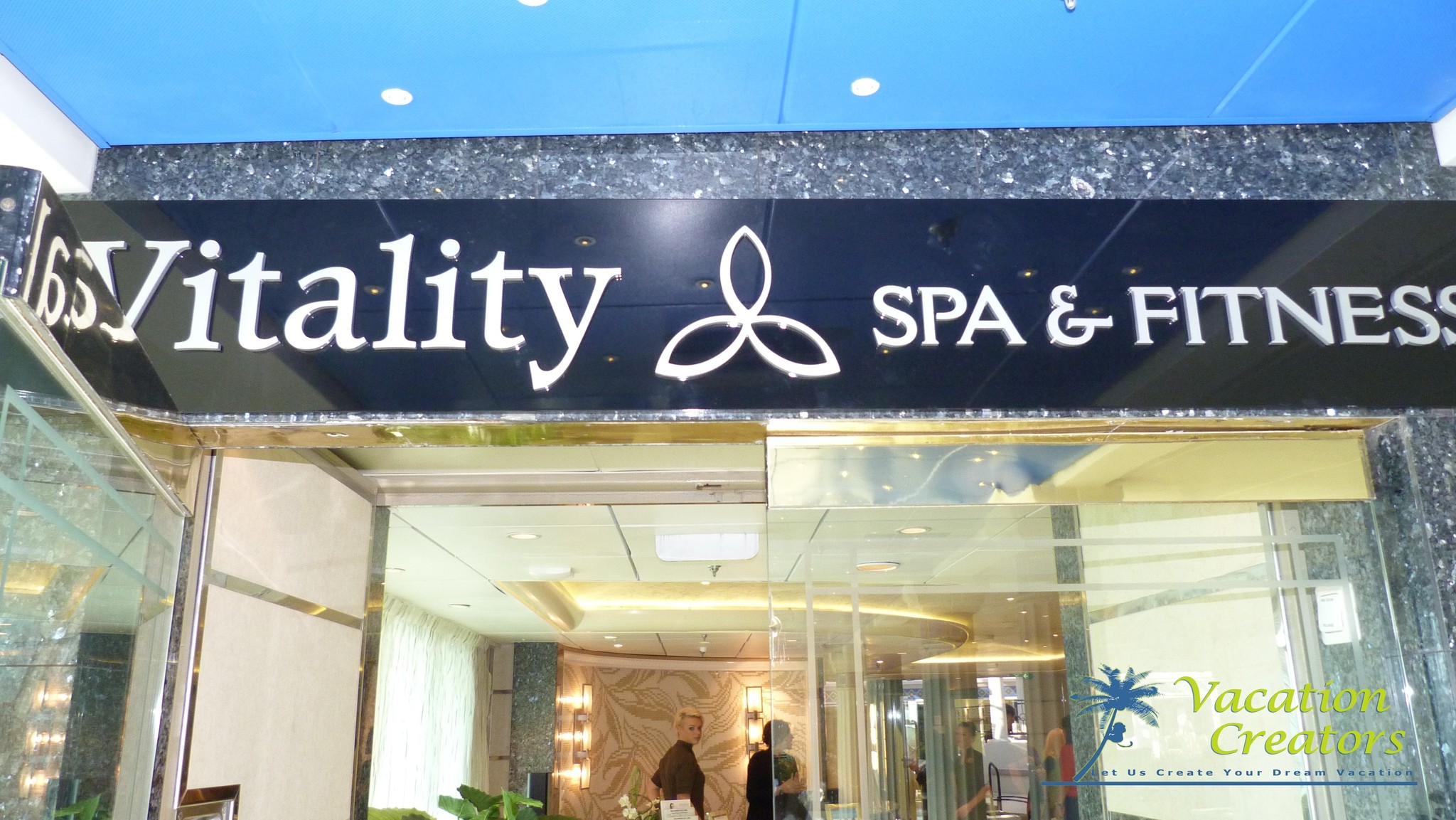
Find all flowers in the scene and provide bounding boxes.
[619,791,660,820]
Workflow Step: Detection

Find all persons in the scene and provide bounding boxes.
[670,803,674,809]
[985,704,1016,743]
[646,707,706,820]
[746,719,805,820]
[985,715,1079,820]
[909,721,993,820]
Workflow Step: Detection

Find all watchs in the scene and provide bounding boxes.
[778,787,784,795]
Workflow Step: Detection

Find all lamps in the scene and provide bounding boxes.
[579,685,592,790]
[746,687,764,757]
